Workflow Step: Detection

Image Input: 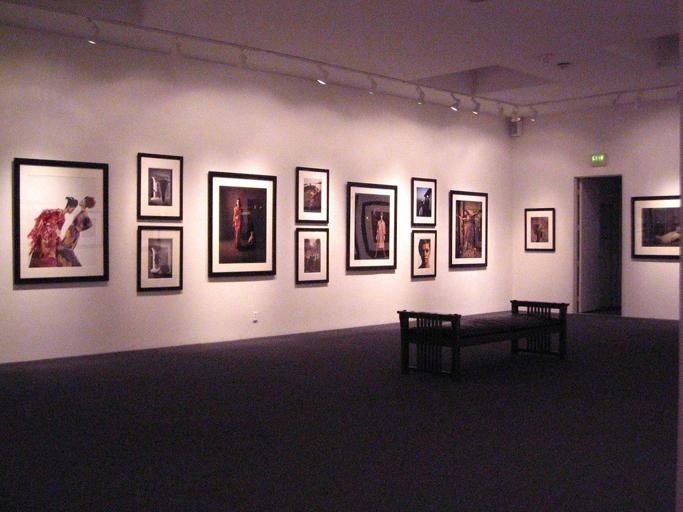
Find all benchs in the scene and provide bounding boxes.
[398,301,569,378]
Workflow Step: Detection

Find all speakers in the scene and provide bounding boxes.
[509,117,522,135]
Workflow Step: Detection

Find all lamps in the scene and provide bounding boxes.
[317,65,329,87]
[367,63,537,123]
[86,18,100,45]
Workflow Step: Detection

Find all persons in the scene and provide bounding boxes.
[60,195,97,267]
[27,195,80,268]
[373,211,388,259]
[232,197,243,249]
[418,239,432,268]
[458,210,478,251]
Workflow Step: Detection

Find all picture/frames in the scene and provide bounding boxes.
[294,229,330,284]
[138,152,183,222]
[524,208,555,252]
[207,170,277,276]
[411,177,438,227]
[11,157,110,282]
[448,189,488,268]
[295,166,329,225]
[137,227,183,290]
[411,229,437,279]
[345,182,398,269]
[632,196,681,261]
